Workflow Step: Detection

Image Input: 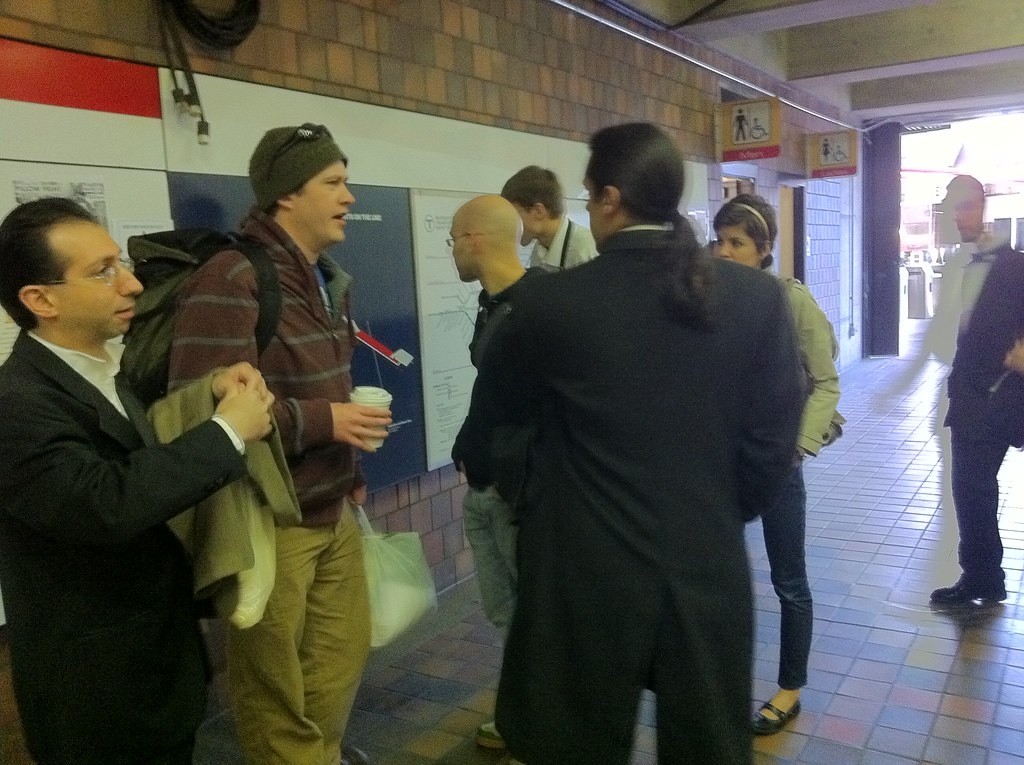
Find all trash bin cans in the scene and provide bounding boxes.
[905,264,947,320]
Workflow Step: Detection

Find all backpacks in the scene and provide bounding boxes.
[119,228,283,416]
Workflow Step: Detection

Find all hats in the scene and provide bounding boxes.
[249,123,348,212]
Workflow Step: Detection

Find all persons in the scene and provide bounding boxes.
[930,175,1024,602]
[0,198,275,765]
[712,193,841,732]
[501,165,600,273]
[167,123,392,765]
[445,194,538,750]
[451,121,803,765]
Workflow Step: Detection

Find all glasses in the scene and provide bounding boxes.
[270,122,332,169]
[446,233,484,247]
[42,258,135,286]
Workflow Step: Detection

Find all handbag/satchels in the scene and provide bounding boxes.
[351,503,437,648]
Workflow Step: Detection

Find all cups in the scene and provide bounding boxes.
[350,386,392,449]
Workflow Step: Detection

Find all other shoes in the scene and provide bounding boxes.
[338,746,368,765]
[930,570,1007,604]
[475,719,508,748]
[751,699,800,735]
